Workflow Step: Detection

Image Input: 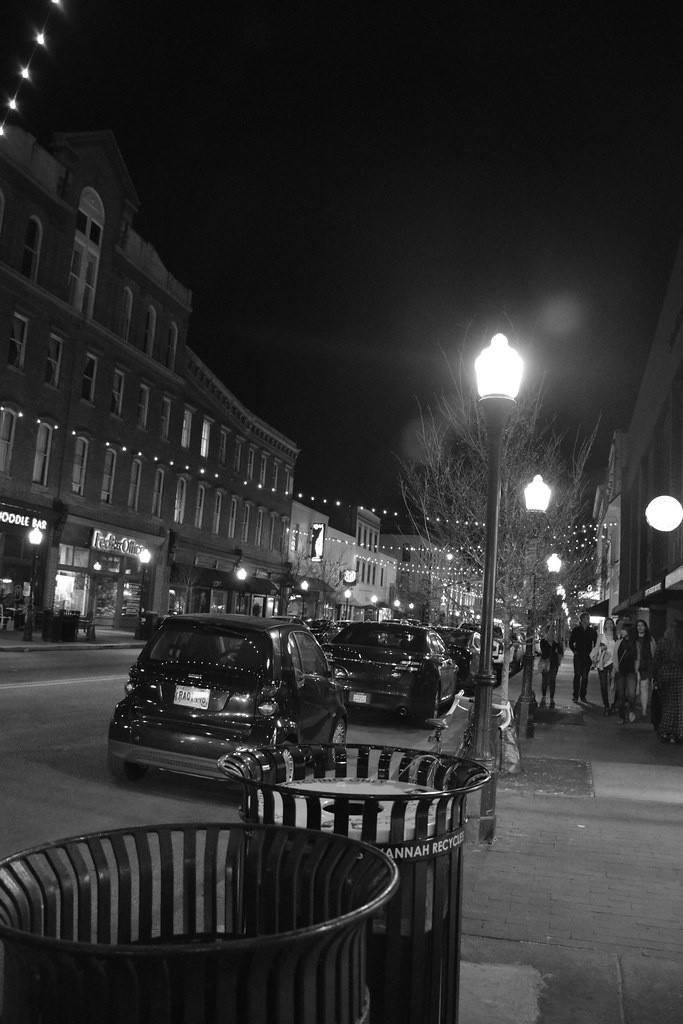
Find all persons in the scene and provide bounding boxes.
[571,615,598,702]
[540,626,563,705]
[592,616,683,743]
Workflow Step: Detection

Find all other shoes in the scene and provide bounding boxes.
[572,697,578,702]
[643,710,648,717]
[580,696,586,701]
[609,709,612,715]
[604,712,608,717]
[615,717,624,724]
[541,697,546,704]
[669,734,678,744]
[550,698,555,705]
[660,731,668,739]
[629,712,635,722]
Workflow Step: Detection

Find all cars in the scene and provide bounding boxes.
[106,613,350,789]
[320,621,459,730]
[426,617,541,692]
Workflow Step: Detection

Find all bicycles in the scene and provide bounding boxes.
[408,689,514,784]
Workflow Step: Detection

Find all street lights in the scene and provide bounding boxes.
[237,568,247,614]
[466,333,525,777]
[547,553,572,649]
[301,579,309,620]
[371,594,377,621]
[23,525,43,641]
[394,599,401,620]
[344,589,351,620]
[410,602,414,620]
[513,473,552,740]
[137,548,152,613]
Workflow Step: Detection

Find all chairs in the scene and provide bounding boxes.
[0,603,95,641]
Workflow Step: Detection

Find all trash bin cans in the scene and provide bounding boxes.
[216,743,492,1024]
[142,611,158,641]
[58,609,81,643]
[0,821,400,1024]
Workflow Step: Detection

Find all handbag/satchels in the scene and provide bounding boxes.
[538,658,550,673]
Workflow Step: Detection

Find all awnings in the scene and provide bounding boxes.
[291,575,335,592]
